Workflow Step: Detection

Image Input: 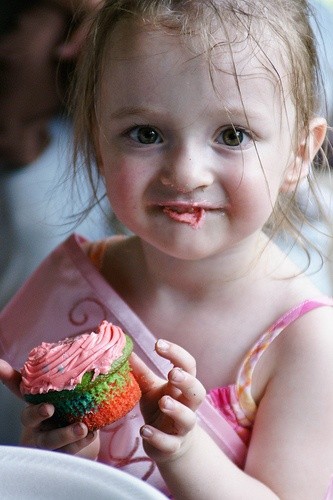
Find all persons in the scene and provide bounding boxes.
[1,1,137,448]
[0,0,333,500]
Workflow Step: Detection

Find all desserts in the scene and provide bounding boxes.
[19,319,143,434]
